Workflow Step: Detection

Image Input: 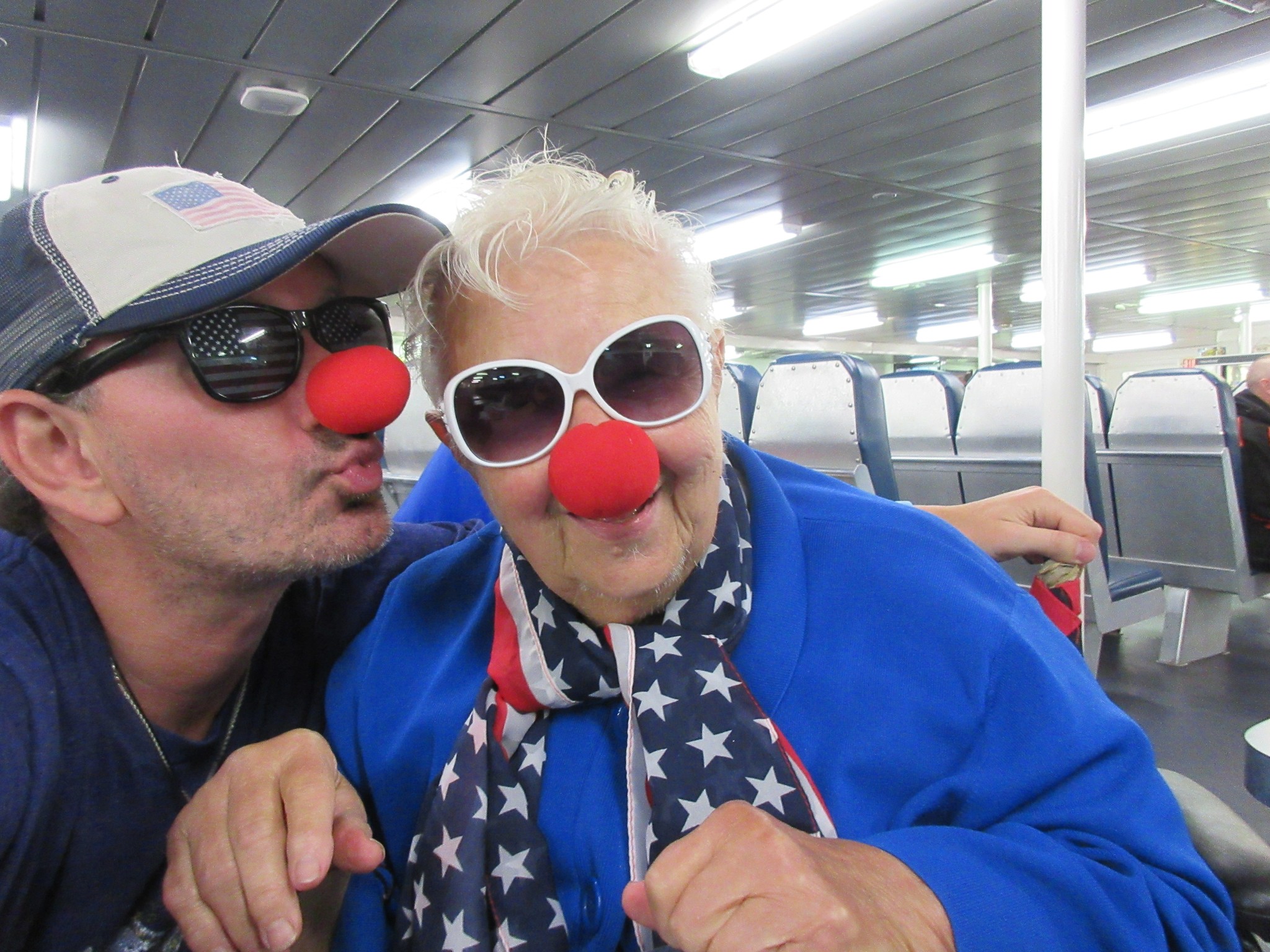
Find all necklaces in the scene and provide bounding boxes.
[110,654,250,802]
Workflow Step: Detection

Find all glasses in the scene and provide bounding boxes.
[30,296,393,403]
[438,314,712,469]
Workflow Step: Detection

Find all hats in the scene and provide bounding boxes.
[0,166,455,399]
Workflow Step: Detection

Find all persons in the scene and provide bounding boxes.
[161,122,1240,952]
[0,166,1102,952]
[1234,357,1270,572]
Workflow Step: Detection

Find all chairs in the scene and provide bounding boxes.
[697,350,1270,697]
